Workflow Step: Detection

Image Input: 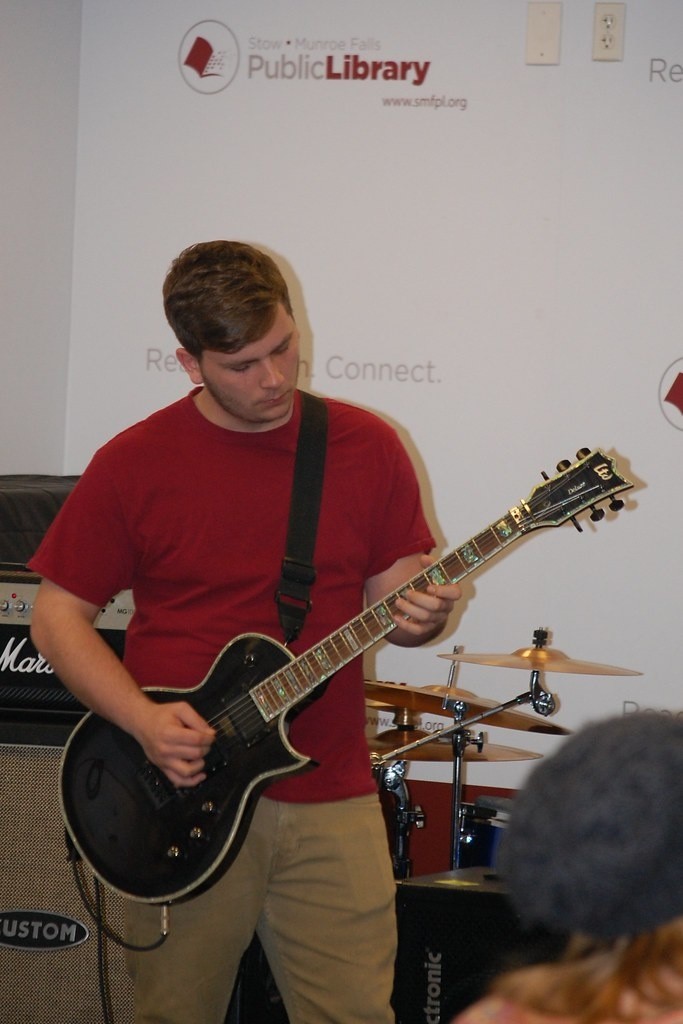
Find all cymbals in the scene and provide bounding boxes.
[366,729,543,762]
[433,648,645,677]
[363,680,573,737]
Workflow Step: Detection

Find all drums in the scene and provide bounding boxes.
[458,802,515,869]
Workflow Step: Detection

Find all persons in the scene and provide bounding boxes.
[453,712,683,1024]
[26,241,463,1024]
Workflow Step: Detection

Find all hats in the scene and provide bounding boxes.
[503,714,681,938]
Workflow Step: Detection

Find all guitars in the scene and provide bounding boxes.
[55,447,634,908]
